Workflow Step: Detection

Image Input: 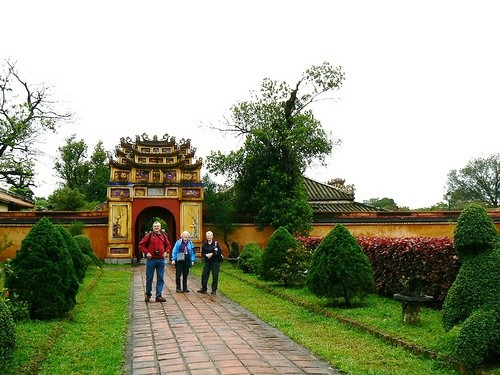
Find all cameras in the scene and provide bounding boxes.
[154,250,160,255]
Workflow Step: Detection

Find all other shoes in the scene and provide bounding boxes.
[211,290,216,295]
[176,289,184,293]
[156,296,166,302]
[197,289,207,293]
[145,293,152,302]
[183,289,190,292]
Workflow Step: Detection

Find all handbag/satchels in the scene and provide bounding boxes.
[219,253,224,262]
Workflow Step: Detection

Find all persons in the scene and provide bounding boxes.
[197,231,222,295]
[139,221,171,302]
[171,231,196,293]
